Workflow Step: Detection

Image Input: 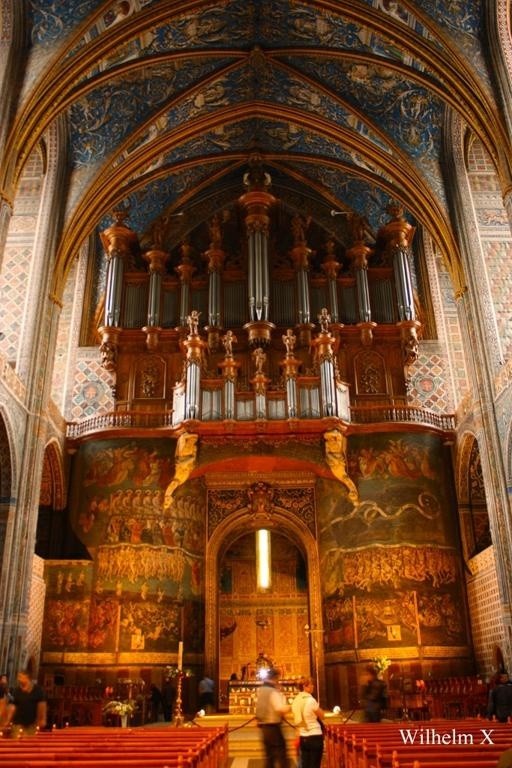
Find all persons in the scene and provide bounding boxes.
[282,328,296,352]
[222,329,234,356]
[255,668,293,767]
[198,672,219,712]
[292,676,343,768]
[187,308,200,336]
[40,437,470,651]
[150,684,160,721]
[318,308,332,333]
[357,665,388,721]
[160,678,174,722]
[4,669,49,732]
[0,674,16,720]
[253,346,266,371]
[486,669,512,723]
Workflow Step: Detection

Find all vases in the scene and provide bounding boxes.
[121,713,128,728]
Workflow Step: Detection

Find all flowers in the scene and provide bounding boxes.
[101,700,139,719]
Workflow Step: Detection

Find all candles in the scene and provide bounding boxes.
[177,641,184,671]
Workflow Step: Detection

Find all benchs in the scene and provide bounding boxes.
[0,722,231,768]
[323,712,512,766]
